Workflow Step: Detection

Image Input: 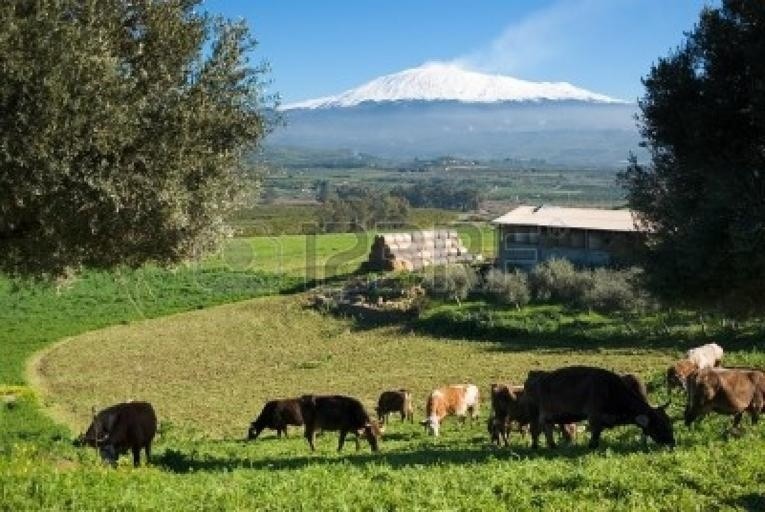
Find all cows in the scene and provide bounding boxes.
[522,365,676,449]
[667,340,724,396]
[418,383,480,437]
[374,388,414,425]
[248,398,324,441]
[487,382,576,449]
[684,360,765,434]
[297,394,381,451]
[81,401,157,469]
